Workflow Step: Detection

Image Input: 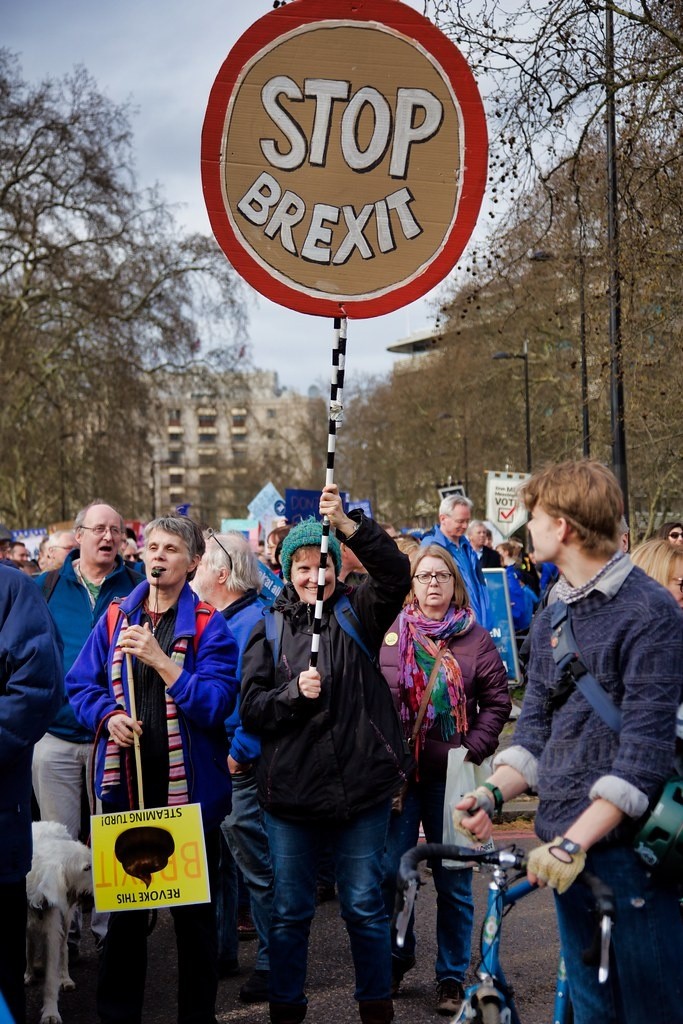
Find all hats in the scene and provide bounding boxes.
[282,517,341,581]
[660,523,683,541]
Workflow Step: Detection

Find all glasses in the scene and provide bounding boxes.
[415,572,452,584]
[671,576,683,592]
[53,545,71,553]
[82,526,121,537]
[207,532,232,571]
[669,532,683,539]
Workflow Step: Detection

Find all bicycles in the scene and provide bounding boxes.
[392,843,613,1024]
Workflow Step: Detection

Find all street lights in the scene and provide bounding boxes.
[437,413,469,498]
[59,431,77,523]
[492,352,532,474]
[527,249,589,459]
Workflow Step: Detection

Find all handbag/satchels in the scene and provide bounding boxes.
[391,788,403,816]
[441,744,495,868]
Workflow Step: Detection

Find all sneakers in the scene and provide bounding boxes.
[434,979,466,1013]
[390,947,416,975]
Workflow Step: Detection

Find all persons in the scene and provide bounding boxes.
[618,514,683,611]
[452,462,683,1024]
[238,484,411,1024]
[252,519,370,585]
[0,504,268,1024]
[376,495,560,720]
[378,545,512,1014]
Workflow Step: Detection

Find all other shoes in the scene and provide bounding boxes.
[235,913,258,939]
[238,971,273,1004]
[313,884,335,903]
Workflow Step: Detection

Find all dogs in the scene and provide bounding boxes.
[23,821,94,1024]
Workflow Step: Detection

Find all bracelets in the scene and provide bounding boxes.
[482,782,504,816]
